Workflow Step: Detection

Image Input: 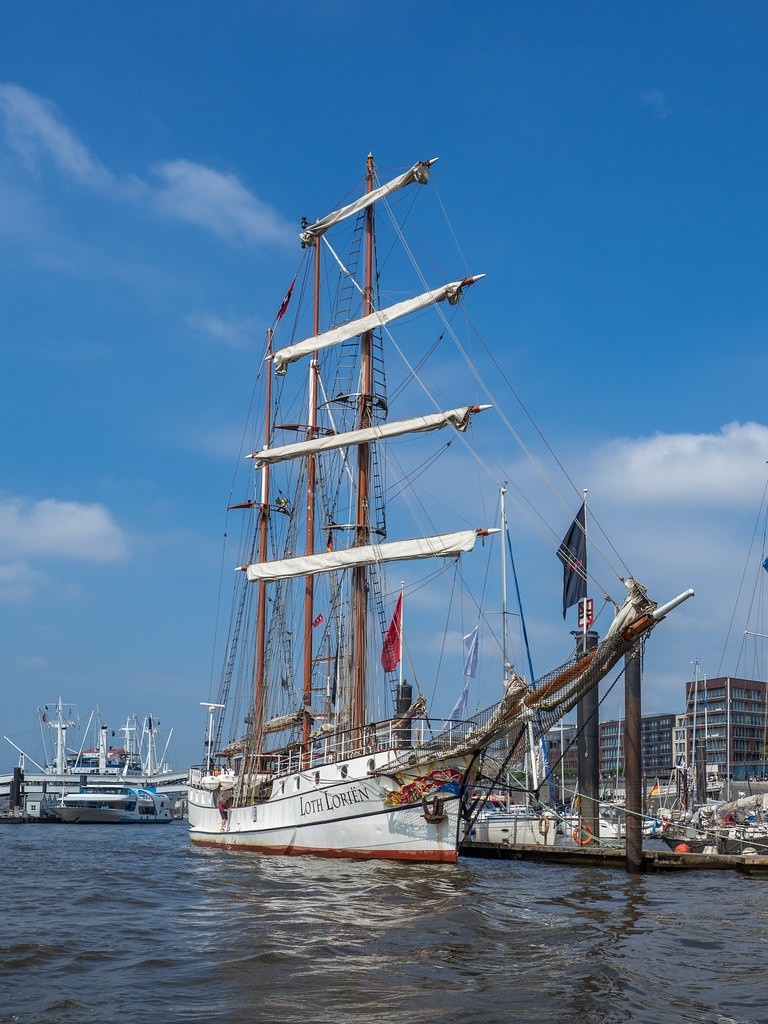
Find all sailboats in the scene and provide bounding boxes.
[186,150,490,860]
[560,484,663,840]
[462,479,559,847]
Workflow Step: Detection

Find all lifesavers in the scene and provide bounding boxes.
[539,817,549,836]
[661,818,668,830]
[574,825,592,844]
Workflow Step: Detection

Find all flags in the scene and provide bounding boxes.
[312,613,324,628]
[555,498,586,623]
[463,625,480,679]
[326,532,334,551]
[282,677,287,690]
[381,590,402,672]
[332,648,338,707]
[647,780,659,800]
[443,683,470,731]
[42,711,47,722]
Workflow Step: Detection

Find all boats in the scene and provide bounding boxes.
[48,785,173,825]
[659,832,768,858]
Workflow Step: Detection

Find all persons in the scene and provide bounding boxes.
[219,800,228,831]
